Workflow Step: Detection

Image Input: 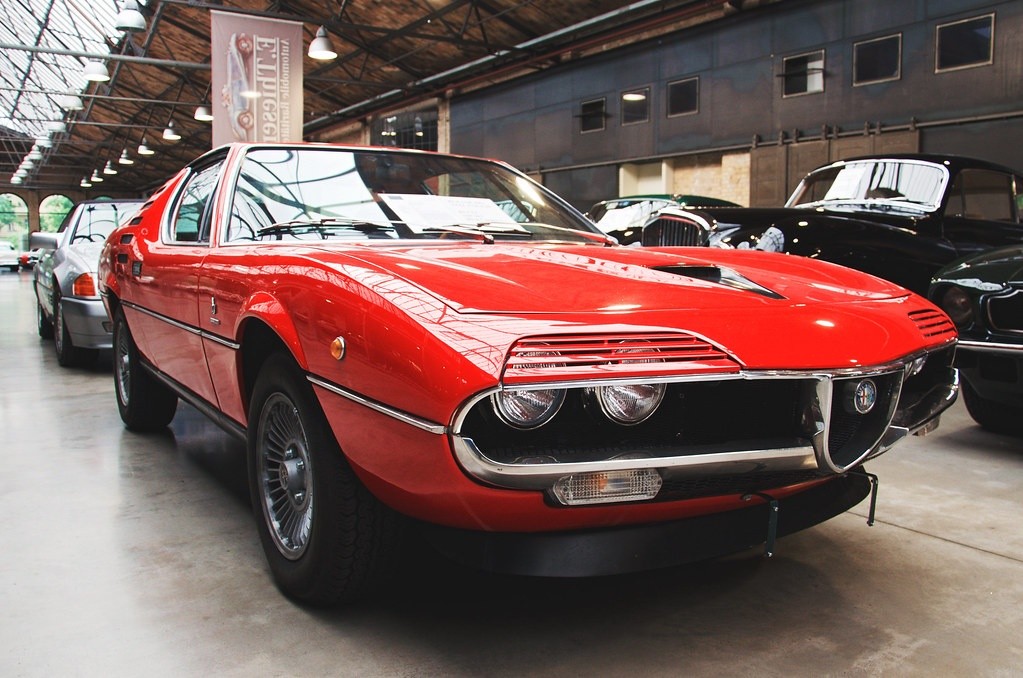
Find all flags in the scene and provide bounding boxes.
[210,9,304,152]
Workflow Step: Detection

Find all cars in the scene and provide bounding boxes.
[27,197,200,372]
[639,152,1023,290]
[929,243,1023,434]
[580,190,742,247]
[97,141,961,615]
